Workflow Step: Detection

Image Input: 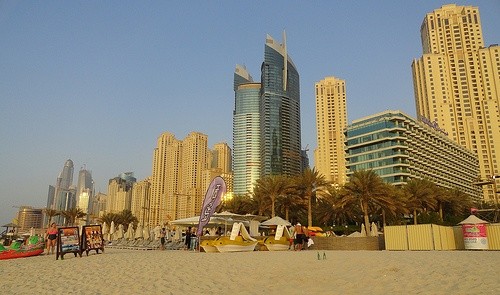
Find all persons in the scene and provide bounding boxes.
[45,223,58,255]
[159,228,166,250]
[167,226,240,244]
[184,226,192,249]
[287,222,309,251]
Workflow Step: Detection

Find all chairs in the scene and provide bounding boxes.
[104,239,189,250]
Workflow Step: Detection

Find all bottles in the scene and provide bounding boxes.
[318,252,320,260]
[323,252,326,259]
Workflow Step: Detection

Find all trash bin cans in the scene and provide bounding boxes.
[190,237,198,250]
